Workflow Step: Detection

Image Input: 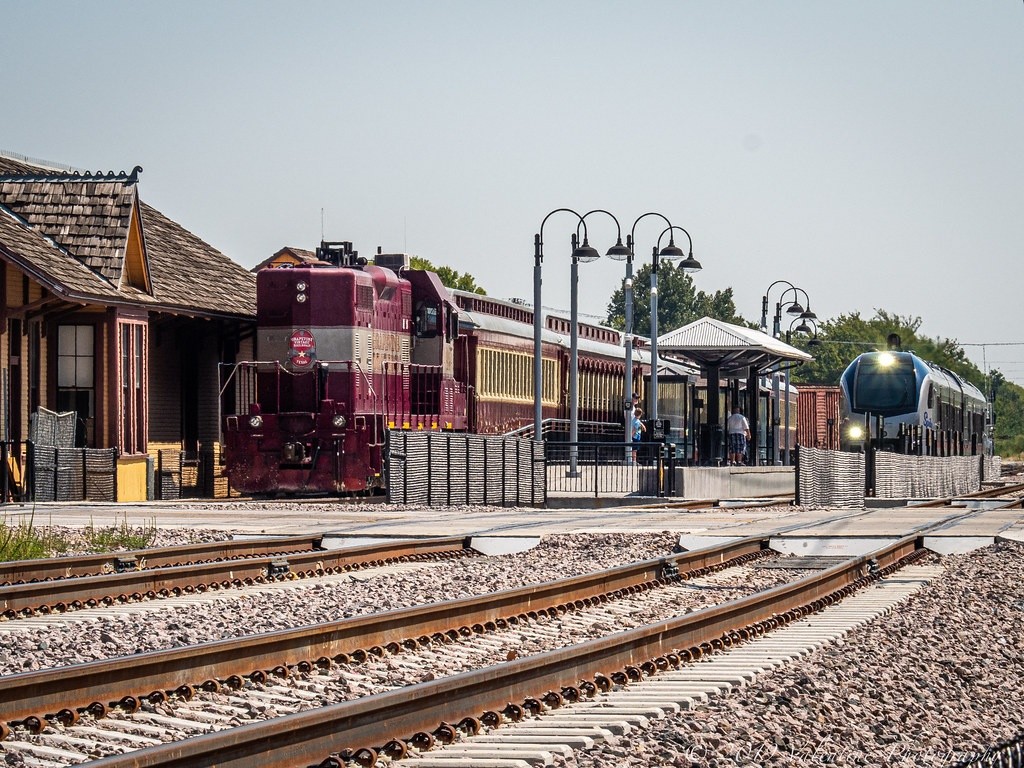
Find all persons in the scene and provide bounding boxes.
[719,403,760,467]
[981,433,987,441]
[936,420,941,430]
[624,393,646,465]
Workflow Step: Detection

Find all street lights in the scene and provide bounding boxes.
[784,318,823,464]
[570,210,632,474]
[532,208,602,443]
[624,213,686,463]
[650,226,703,421]
[760,280,804,464]
[773,288,817,465]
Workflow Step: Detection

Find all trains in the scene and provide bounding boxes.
[835,332,994,485]
[219,206,801,501]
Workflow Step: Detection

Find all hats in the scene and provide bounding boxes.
[632,393,641,398]
[635,408,645,415]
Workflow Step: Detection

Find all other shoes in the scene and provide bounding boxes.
[738,461,746,466]
[731,461,737,466]
